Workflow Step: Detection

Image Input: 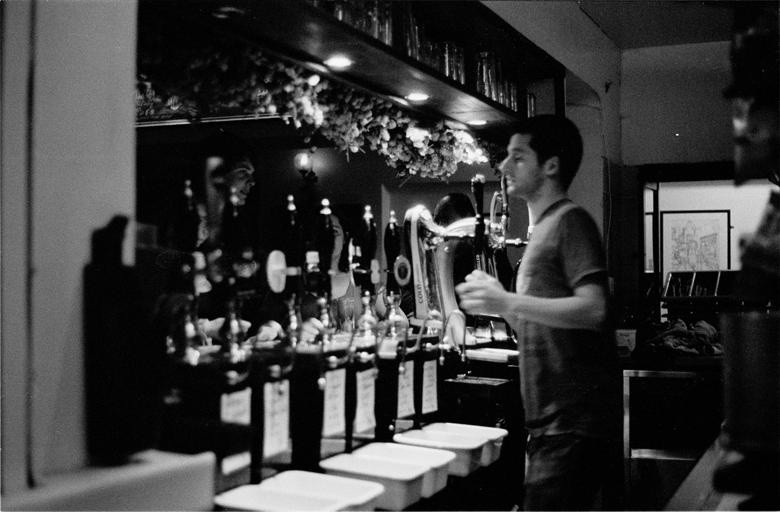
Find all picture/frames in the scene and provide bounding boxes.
[660,209,732,288]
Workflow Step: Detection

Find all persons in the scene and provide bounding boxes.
[226,144,260,207]
[453,114,616,511]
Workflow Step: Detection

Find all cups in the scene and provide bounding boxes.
[615,329,637,352]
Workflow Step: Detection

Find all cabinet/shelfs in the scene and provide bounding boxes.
[620,367,730,465]
